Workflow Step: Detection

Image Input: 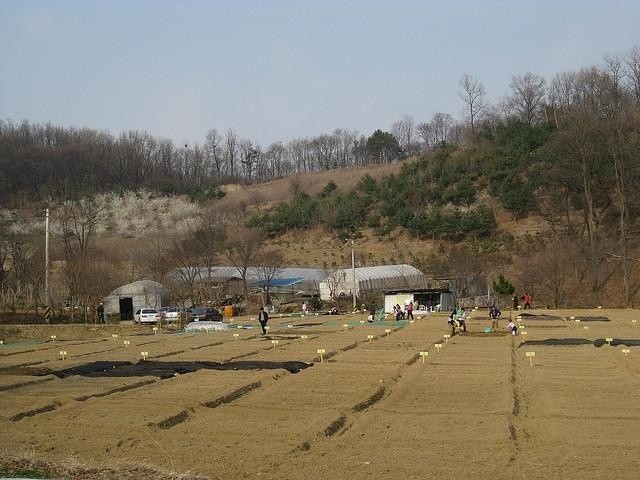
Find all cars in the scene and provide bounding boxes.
[134,307,223,325]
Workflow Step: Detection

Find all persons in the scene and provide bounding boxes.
[301,301,307,314]
[369,301,375,320]
[258,307,268,335]
[447,308,457,337]
[367,313,375,323]
[488,291,533,336]
[392,299,419,321]
[96,302,105,325]
[457,307,467,332]
